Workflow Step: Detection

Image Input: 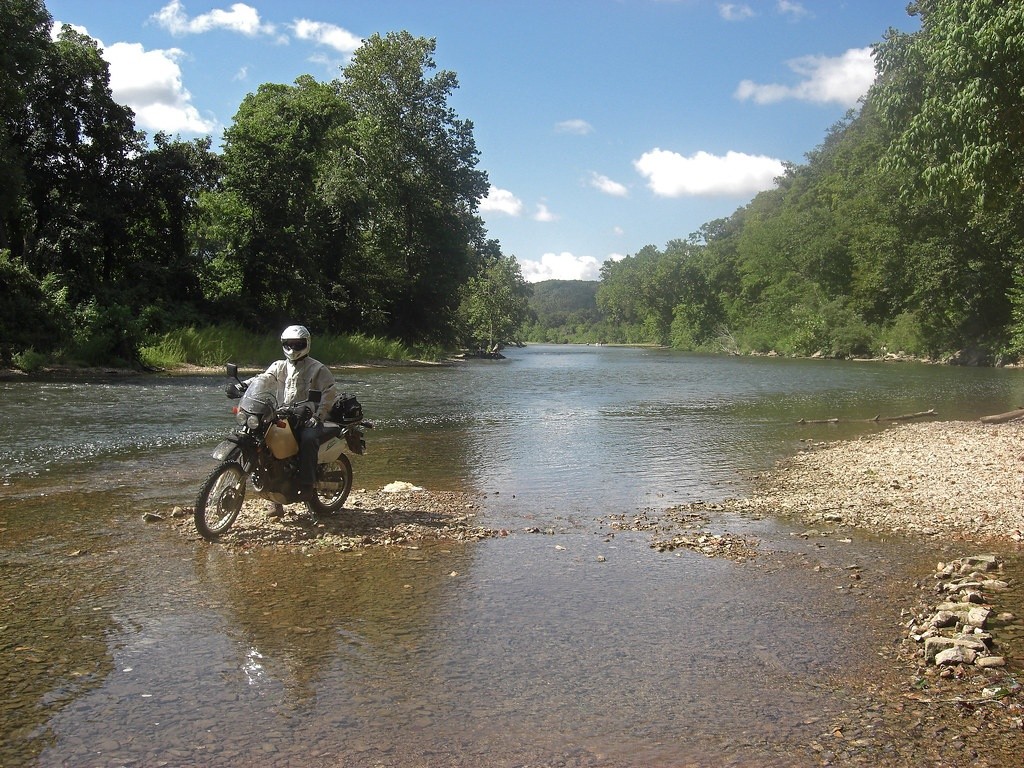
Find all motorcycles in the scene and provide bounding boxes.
[194,362,373,539]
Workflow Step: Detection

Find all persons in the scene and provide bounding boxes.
[225,326,335,517]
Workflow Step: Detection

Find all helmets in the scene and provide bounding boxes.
[280,324,312,361]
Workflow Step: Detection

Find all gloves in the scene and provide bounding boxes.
[304,413,323,428]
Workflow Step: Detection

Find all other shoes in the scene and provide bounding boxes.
[302,485,313,498]
[266,508,284,517]
